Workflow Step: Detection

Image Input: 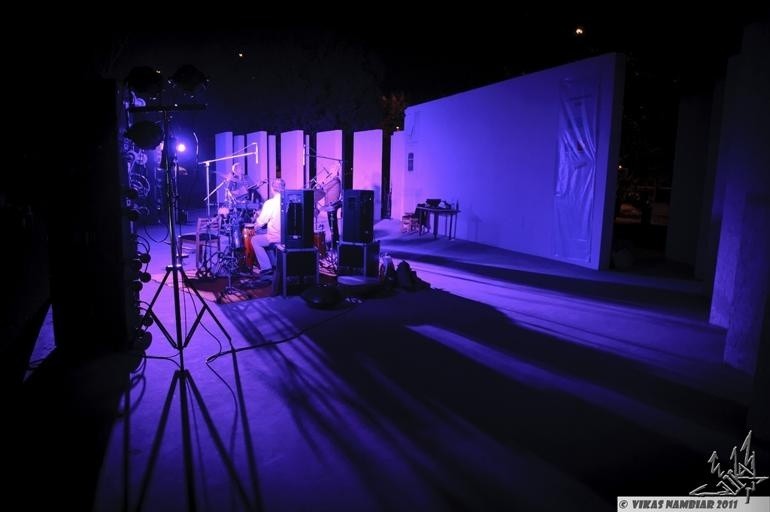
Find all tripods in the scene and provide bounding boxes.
[217,231,252,304]
[137,109,232,352]
[195,218,226,275]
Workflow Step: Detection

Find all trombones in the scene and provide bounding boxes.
[300,167,333,192]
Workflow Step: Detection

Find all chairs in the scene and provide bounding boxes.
[402,203,425,233]
[178,215,222,272]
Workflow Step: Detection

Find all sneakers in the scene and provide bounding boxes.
[259,268,273,275]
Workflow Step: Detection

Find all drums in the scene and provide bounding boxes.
[313,230,327,260]
[234,223,256,273]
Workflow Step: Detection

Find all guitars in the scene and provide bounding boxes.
[248,180,266,191]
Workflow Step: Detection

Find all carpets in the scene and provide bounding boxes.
[149,266,276,305]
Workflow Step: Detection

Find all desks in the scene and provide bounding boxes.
[417,203,459,240]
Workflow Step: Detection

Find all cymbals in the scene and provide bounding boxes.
[313,191,326,205]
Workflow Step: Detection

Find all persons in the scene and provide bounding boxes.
[321,163,341,248]
[250,177,287,276]
[225,161,255,227]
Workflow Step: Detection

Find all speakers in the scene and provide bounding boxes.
[342,189,375,243]
[281,190,314,248]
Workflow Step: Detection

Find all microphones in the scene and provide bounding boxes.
[302,148,305,166]
[256,145,259,163]
